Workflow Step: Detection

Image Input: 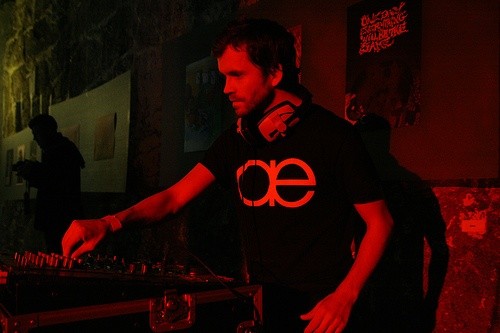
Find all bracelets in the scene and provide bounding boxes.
[101,215,124,239]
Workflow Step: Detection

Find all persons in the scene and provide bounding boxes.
[60,16,395,333]
[12,112,84,256]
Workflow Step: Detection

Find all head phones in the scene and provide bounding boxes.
[237,84,312,145]
